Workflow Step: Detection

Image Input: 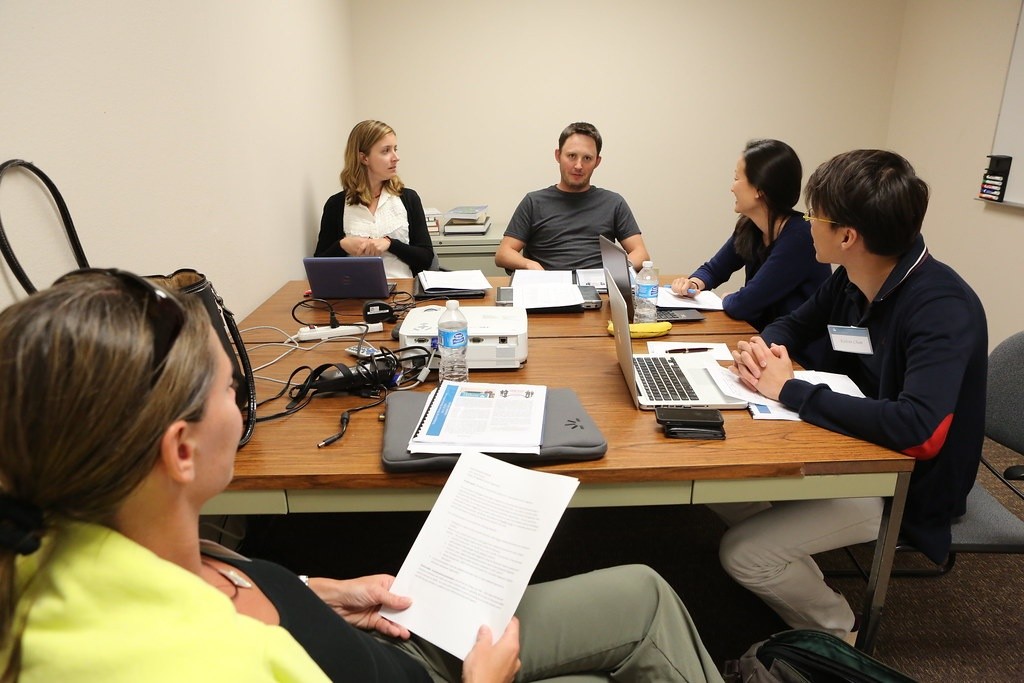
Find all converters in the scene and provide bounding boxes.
[315,361,392,394]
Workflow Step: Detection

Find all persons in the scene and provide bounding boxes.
[313,119,434,279]
[670,140,831,331]
[0,268,726,683]
[698,148,988,644]
[496,122,650,272]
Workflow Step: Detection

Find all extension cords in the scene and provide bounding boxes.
[298,321,384,341]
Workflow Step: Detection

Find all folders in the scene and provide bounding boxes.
[413,274,484,300]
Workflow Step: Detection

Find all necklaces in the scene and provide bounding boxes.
[201,560,251,598]
[375,195,380,199]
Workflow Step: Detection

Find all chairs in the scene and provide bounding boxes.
[823,330,1024,658]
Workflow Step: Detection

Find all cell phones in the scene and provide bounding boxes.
[655,407,724,427]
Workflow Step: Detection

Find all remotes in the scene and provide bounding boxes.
[345,345,399,362]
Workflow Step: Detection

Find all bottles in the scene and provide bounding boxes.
[633,259,658,323]
[436,299,470,386]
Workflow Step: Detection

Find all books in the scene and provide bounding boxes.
[443,205,492,235]
[748,403,801,421]
[425,216,439,236]
[411,379,547,448]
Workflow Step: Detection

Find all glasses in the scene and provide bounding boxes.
[51,268,187,388]
[803,207,844,225]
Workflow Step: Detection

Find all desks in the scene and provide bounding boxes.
[209,277,916,656]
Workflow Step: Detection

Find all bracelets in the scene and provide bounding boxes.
[692,281,699,289]
[298,575,309,586]
[385,235,394,244]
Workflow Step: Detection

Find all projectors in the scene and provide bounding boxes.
[398,305,529,369]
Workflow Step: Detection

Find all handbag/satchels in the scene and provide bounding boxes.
[718,629,920,683]
[0,159,257,449]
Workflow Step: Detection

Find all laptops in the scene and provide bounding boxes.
[598,234,749,410]
[494,285,602,309]
[302,257,397,299]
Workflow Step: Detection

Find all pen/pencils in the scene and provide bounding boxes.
[664,285,696,293]
[665,348,714,353]
